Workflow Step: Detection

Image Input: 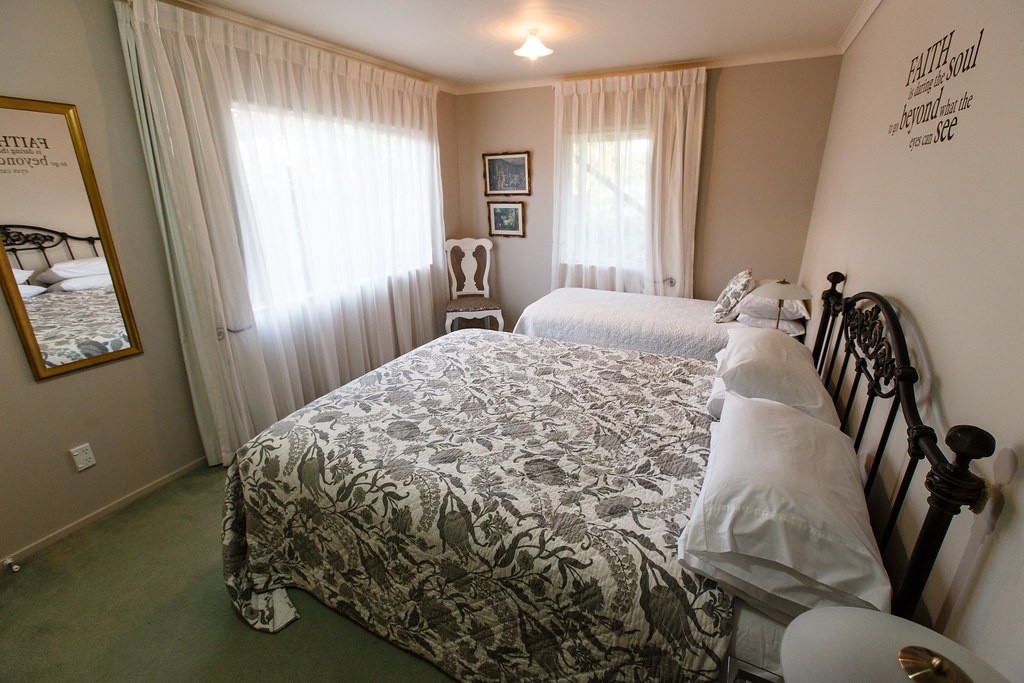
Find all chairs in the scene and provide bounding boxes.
[443,238,505,335]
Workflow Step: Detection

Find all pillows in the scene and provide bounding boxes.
[35,256,110,284]
[11,268,35,285]
[705,348,729,420]
[686,390,894,618]
[47,274,114,293]
[17,285,48,300]
[716,328,841,430]
[712,269,755,324]
[736,312,806,338]
[737,278,810,320]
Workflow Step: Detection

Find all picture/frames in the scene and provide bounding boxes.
[487,200,525,238]
[481,150,531,197]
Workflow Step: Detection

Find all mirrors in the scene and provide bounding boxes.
[0,95,144,383]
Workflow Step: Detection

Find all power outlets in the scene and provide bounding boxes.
[69,442,97,473]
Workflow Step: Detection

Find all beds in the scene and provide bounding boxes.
[221,271,997,683]
[512,286,753,362]
[0,224,131,369]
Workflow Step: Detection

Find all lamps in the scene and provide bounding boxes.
[751,277,814,330]
[779,607,1013,683]
[513,28,553,62]
